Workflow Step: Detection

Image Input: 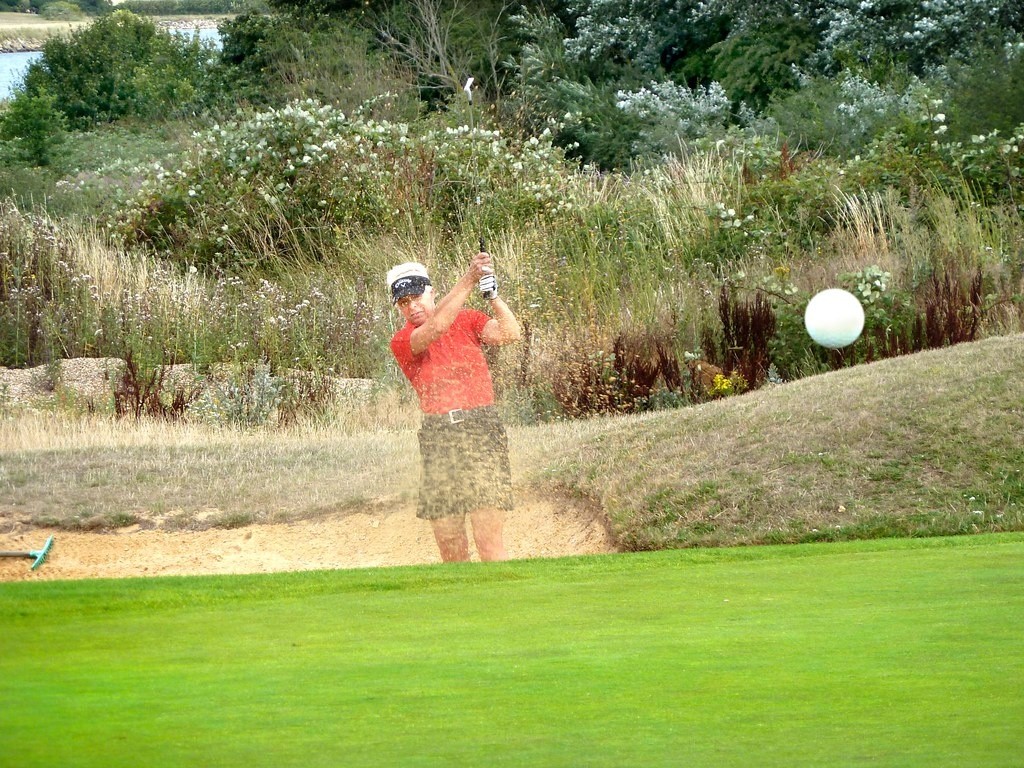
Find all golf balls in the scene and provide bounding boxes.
[804,288,866,349]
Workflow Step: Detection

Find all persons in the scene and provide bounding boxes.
[386,252,522,563]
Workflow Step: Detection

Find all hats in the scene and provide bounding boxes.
[387,262,433,307]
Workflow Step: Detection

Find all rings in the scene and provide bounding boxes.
[481,266,491,274]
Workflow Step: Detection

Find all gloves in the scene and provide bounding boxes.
[479,274,498,300]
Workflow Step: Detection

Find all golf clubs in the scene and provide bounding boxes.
[463,77,491,299]
